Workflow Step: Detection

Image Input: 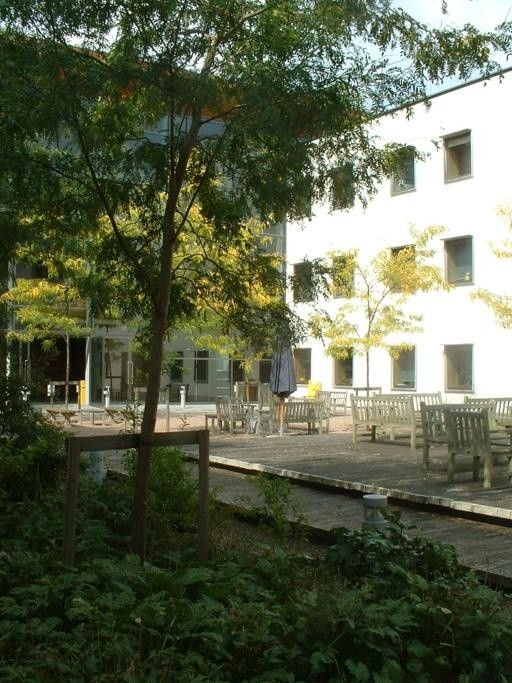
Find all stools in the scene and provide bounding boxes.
[45,406,125,426]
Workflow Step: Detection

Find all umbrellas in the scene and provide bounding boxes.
[269,320,297,437]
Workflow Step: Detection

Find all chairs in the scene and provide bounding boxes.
[204,393,259,434]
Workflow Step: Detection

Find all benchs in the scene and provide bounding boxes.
[267,387,512,489]
